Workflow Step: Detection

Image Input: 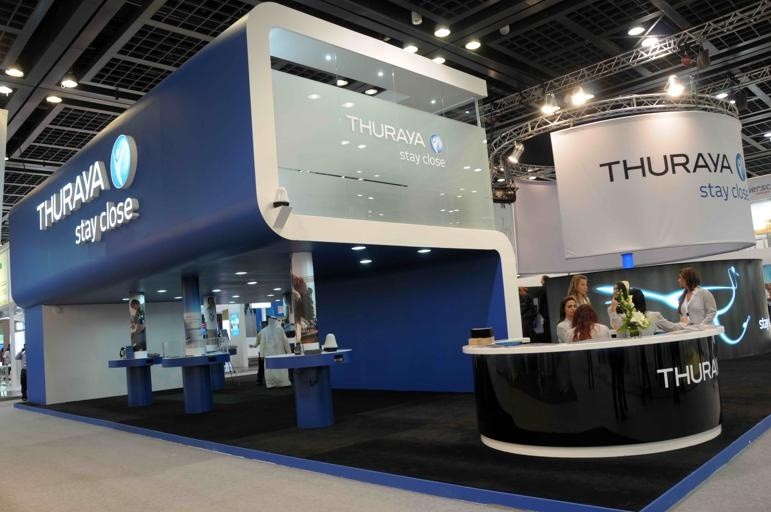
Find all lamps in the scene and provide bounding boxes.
[506,139,526,165]
[662,74,685,98]
[432,22,451,38]
[539,84,585,115]
[59,71,79,89]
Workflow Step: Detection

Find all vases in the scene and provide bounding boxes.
[626,326,643,341]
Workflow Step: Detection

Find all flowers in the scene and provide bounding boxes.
[610,288,650,336]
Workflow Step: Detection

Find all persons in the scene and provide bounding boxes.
[614,288,691,339]
[674,268,718,329]
[291,271,314,324]
[249,321,269,385]
[0,343,10,376]
[260,316,293,391]
[606,280,631,342]
[555,295,577,344]
[518,275,553,343]
[129,298,146,331]
[562,273,594,310]
[14,344,27,401]
[563,304,612,343]
[205,297,217,322]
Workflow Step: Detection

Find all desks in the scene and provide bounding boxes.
[160,353,232,415]
[106,356,163,408]
[263,347,354,430]
[208,352,230,392]
